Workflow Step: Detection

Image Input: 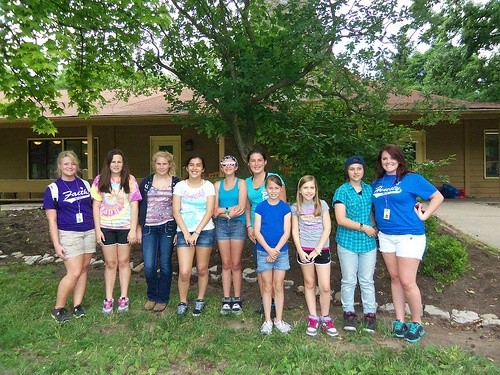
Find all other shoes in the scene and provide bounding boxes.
[258,299,265,314]
[154,303,166,312]
[270,301,276,318]
[144,300,155,310]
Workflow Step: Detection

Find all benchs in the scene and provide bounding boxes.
[0,192,45,203]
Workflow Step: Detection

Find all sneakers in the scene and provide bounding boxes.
[305,317,319,337]
[231,297,242,315]
[220,297,232,316]
[322,315,338,337]
[362,312,376,333]
[175,302,187,314]
[274,319,291,333]
[73,305,85,318]
[51,306,69,323]
[261,321,273,336]
[192,299,204,317]
[405,322,425,343]
[102,298,114,315]
[391,321,407,337]
[342,311,356,332]
[116,296,129,313]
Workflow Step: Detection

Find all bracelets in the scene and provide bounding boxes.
[373,225,377,228]
[314,246,322,256]
[246,225,252,229]
[194,231,200,235]
[358,223,363,232]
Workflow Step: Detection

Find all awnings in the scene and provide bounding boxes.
[225,207,231,220]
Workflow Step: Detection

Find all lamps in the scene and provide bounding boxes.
[184,139,193,151]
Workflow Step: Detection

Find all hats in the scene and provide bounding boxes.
[345,156,366,166]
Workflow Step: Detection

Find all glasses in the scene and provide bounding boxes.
[221,163,236,168]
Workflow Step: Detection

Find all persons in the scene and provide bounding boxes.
[331,155,382,333]
[172,156,216,317]
[254,174,292,336]
[43,151,98,324]
[129,150,184,312]
[287,175,340,338]
[90,150,143,314]
[208,155,247,317]
[371,144,444,343]
[243,149,287,314]
